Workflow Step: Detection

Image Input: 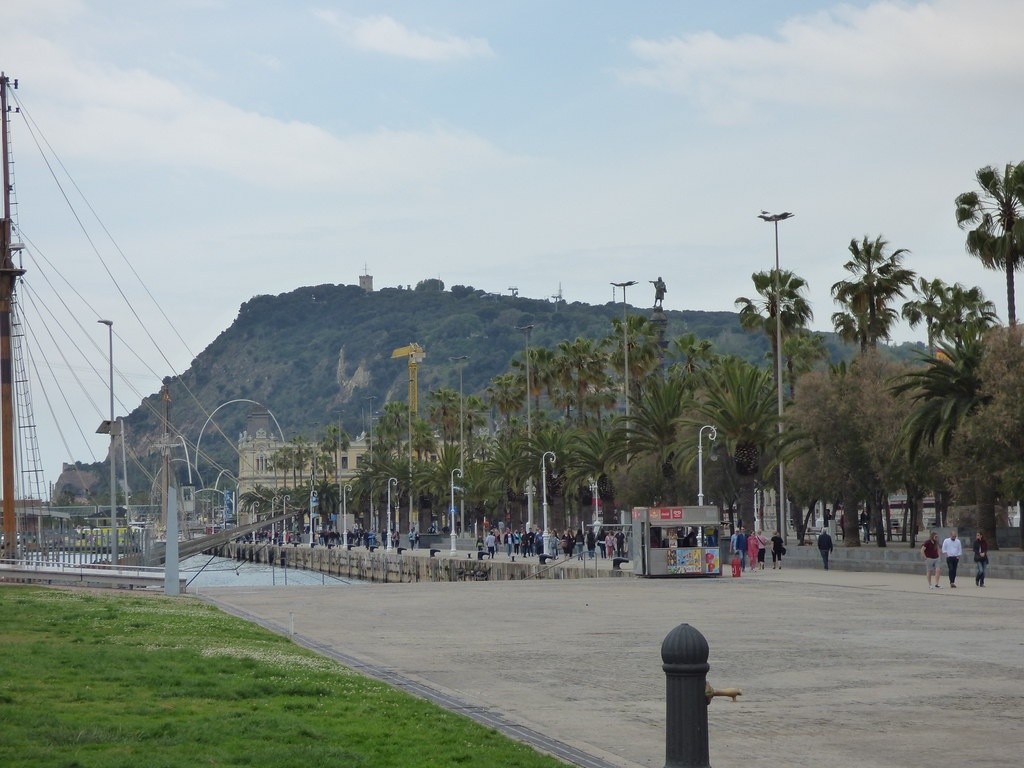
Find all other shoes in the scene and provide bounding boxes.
[935,585,943,589]
[928,586,933,589]
[951,584,956,587]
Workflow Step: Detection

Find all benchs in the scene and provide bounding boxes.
[804,526,822,546]
[888,520,917,541]
[835,521,863,542]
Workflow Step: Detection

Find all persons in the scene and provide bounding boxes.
[860,507,870,544]
[840,509,845,543]
[921,531,941,589]
[973,531,989,587]
[408,529,416,551]
[942,531,962,587]
[730,526,783,572]
[476,519,626,560]
[818,528,833,571]
[682,532,698,547]
[823,509,835,527]
[233,529,292,544]
[381,528,401,551]
[321,524,370,550]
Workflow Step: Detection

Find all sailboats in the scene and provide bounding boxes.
[0,70,308,590]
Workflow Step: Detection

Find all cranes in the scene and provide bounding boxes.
[390,343,427,418]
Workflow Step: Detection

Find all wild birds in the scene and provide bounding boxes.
[761,209,771,216]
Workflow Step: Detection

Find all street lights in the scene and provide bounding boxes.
[365,396,378,531]
[98,320,117,571]
[758,212,796,547]
[515,325,537,533]
[610,280,639,471]
[449,355,471,537]
